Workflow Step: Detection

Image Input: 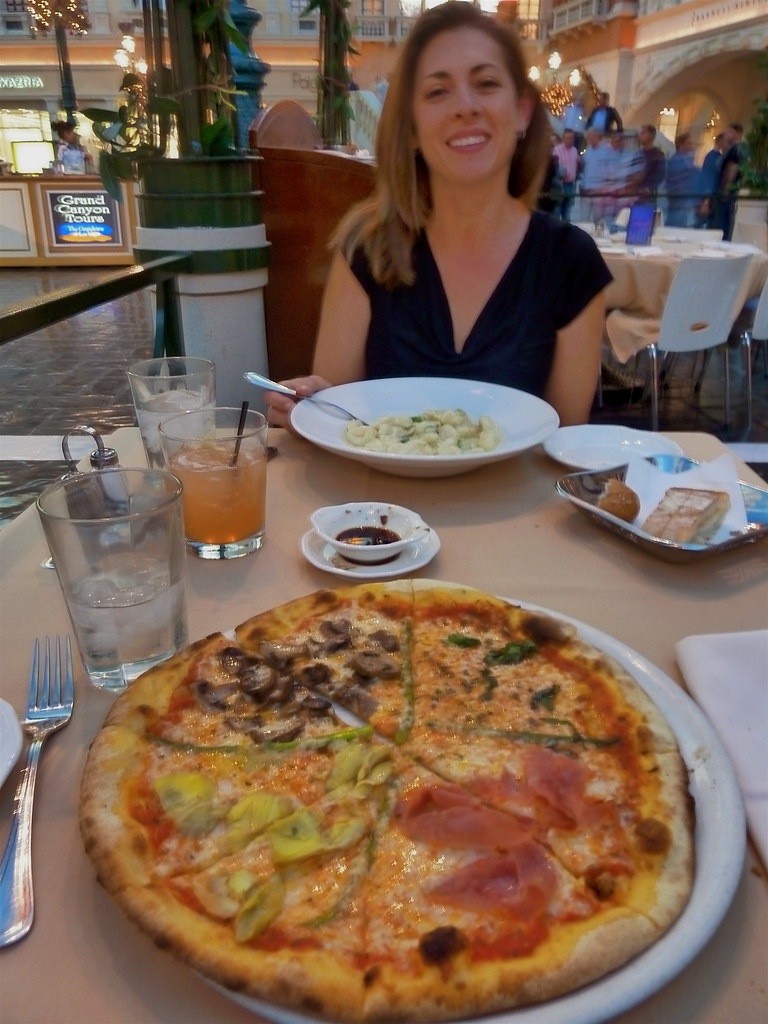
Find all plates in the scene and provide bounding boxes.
[542,423,685,477]
[302,524,442,580]
[596,231,761,258]
[0,699,24,793]
[188,599,745,1023]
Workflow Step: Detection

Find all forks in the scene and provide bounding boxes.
[0,637,76,951]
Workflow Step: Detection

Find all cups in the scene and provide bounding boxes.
[127,357,219,471]
[158,406,269,561]
[35,468,190,694]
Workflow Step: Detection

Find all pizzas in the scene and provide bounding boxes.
[78,577,700,1024]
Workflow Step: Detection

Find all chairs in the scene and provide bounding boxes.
[578,216,768,443]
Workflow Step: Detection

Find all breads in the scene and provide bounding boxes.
[642,487,730,545]
[598,477,640,522]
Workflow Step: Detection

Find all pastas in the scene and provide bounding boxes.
[344,407,497,458]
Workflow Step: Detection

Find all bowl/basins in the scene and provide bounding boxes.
[553,453,767,563]
[311,501,431,563]
[289,378,560,478]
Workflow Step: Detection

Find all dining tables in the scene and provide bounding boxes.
[595,236,767,417]
[0,421,768,1023]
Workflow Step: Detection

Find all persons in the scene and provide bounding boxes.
[57,122,94,172]
[539,92,747,242]
[263,1,613,433]
